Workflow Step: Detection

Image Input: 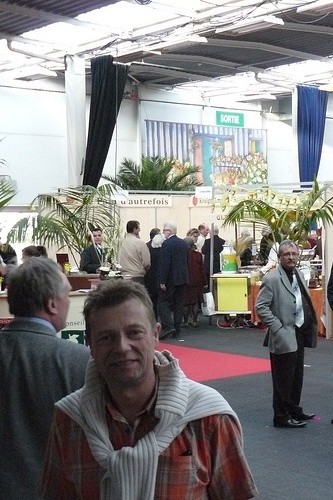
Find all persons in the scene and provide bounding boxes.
[43,281,258,500]
[1,218,322,329]
[1,256,92,500]
[157,224,190,339]
[117,222,152,281]
[255,240,319,428]
[181,238,204,329]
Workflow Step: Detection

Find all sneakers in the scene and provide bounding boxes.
[158,326,176,339]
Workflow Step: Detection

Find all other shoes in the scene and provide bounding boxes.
[192,319,199,326]
[180,320,189,327]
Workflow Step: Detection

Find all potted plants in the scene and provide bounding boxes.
[4,184,129,291]
[205,174,333,335]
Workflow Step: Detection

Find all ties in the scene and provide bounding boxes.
[292,271,305,328]
[99,248,102,255]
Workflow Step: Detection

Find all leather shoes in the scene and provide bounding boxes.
[274,418,307,427]
[293,412,315,419]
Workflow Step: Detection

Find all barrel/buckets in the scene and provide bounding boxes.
[219,245,237,274]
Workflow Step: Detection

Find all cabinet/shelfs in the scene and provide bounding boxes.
[212,272,261,330]
